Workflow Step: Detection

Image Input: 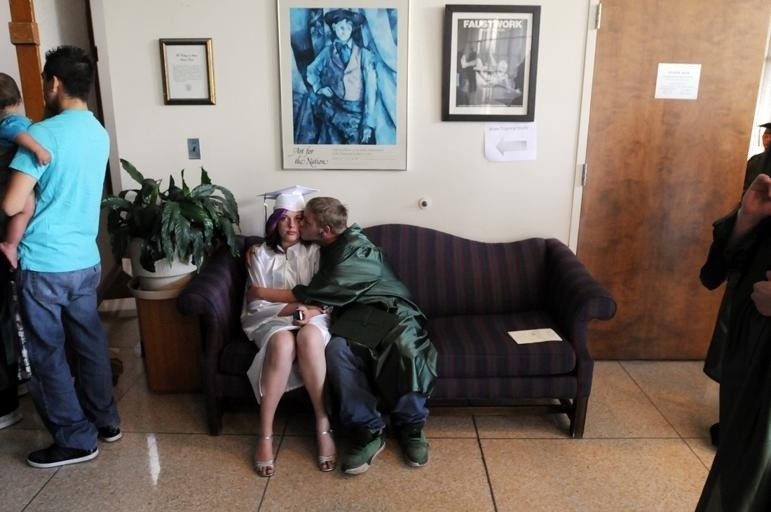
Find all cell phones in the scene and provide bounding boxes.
[293,310,304,321]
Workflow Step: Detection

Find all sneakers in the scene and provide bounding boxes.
[342,436,385,474]
[27,429,122,468]
[400,432,429,467]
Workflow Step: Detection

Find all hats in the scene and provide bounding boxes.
[257,185,319,224]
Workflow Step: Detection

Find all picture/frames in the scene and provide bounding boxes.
[441,4,541,124]
[158,36,219,106]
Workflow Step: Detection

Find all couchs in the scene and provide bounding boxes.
[174,223,619,441]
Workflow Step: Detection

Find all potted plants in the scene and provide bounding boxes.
[100,157,242,291]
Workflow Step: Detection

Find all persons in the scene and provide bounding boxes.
[246,196,432,477]
[1,43,123,469]
[696,123,771,512]
[460,44,511,107]
[247,184,341,474]
[0,73,53,271]
[304,8,382,145]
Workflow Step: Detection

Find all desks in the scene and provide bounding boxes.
[136,294,206,396]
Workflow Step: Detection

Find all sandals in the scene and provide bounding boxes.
[254,435,275,478]
[316,427,337,472]
